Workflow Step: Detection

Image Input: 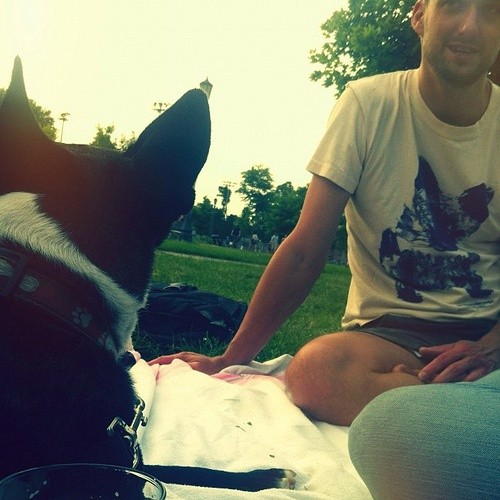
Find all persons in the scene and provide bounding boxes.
[348,366,500,500]
[228,225,290,254]
[146,0,500,426]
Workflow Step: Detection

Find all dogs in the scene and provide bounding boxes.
[0,55,297,500]
